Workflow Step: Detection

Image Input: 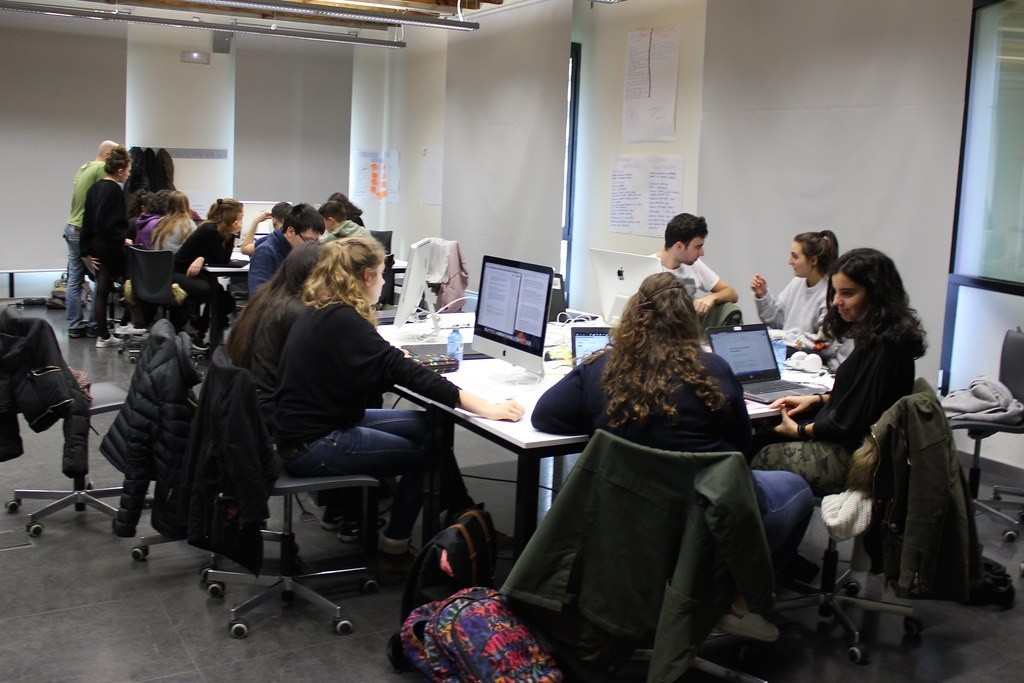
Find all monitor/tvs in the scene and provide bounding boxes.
[471,255,554,386]
[241,201,292,240]
[589,247,664,327]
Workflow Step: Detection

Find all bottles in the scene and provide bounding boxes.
[771,334,787,374]
[446,327,464,366]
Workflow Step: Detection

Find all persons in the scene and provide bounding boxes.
[750,230,839,364]
[175,198,244,360]
[752,248,927,581]
[63,140,119,338]
[79,147,147,348]
[650,213,740,341]
[127,189,197,253]
[227,242,386,541]
[328,192,365,227]
[283,238,514,585]
[318,201,369,242]
[531,272,814,639]
[241,201,291,255]
[249,203,325,297]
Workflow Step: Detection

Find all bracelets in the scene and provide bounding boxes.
[813,393,823,405]
[798,423,810,441]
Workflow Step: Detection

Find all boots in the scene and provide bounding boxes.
[375,528,415,583]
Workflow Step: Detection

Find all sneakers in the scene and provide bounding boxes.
[723,612,780,642]
[114,323,145,335]
[337,518,387,540]
[86,329,98,337]
[68,329,85,337]
[321,509,353,530]
[95,335,122,348]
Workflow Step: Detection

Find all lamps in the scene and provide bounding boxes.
[0,0,481,50]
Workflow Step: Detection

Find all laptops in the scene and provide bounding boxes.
[571,327,616,371]
[706,324,827,405]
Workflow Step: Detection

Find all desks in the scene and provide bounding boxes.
[197,246,255,360]
[367,313,844,568]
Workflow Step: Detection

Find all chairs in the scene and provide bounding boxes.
[0,230,1024,683]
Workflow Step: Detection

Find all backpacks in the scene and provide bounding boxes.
[400,504,496,620]
[47,272,90,310]
[396,584,567,683]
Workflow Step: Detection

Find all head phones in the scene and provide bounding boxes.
[783,351,823,373]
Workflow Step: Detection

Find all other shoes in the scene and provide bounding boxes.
[180,328,210,350]
